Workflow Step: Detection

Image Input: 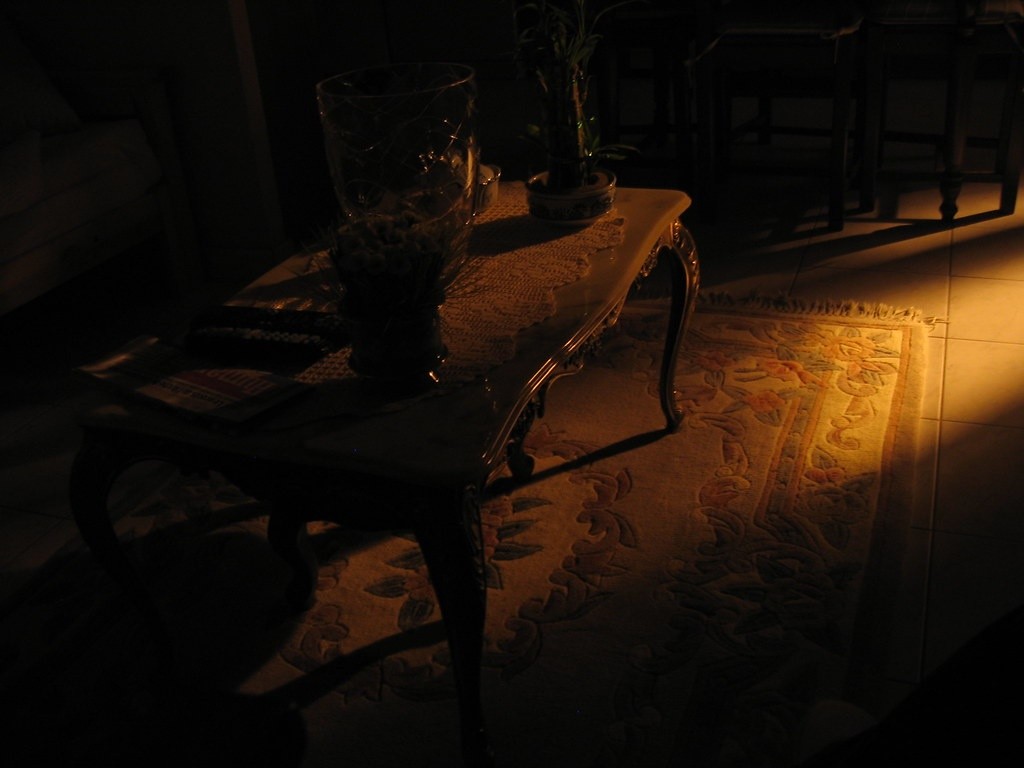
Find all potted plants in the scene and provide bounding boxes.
[500,0,625,227]
[279,185,485,396]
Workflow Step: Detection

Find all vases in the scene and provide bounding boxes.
[315,61,480,283]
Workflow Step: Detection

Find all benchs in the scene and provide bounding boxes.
[0,27,199,315]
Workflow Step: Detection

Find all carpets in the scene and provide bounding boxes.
[0,283,950,768]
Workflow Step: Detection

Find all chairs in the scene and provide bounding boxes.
[585,0,1024,231]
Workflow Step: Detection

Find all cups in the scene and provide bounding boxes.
[316,63,477,289]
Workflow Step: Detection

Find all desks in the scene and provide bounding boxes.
[57,178,703,768]
